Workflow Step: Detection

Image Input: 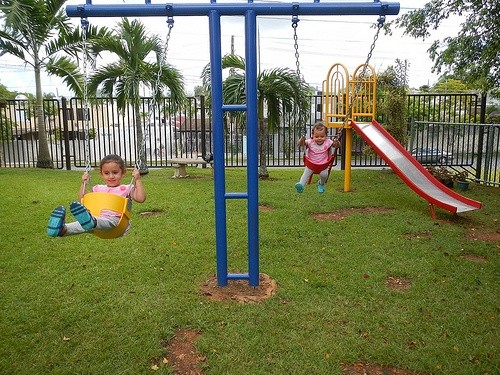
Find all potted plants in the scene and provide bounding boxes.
[426,166,469,191]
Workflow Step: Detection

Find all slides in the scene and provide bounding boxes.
[351,117,484,217]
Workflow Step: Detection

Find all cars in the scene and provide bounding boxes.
[408,147,454,164]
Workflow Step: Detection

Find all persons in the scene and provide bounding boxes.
[294,123,341,193]
[46,154,146,238]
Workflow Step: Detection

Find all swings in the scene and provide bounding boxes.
[289,17,387,182]
[78,14,175,240]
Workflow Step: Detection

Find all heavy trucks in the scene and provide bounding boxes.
[7,105,93,141]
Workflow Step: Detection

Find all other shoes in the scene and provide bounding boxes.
[317,181,325,192]
[294,183,304,193]
[69,201,97,232]
[47,206,67,237]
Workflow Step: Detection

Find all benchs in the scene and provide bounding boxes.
[169,158,214,178]
